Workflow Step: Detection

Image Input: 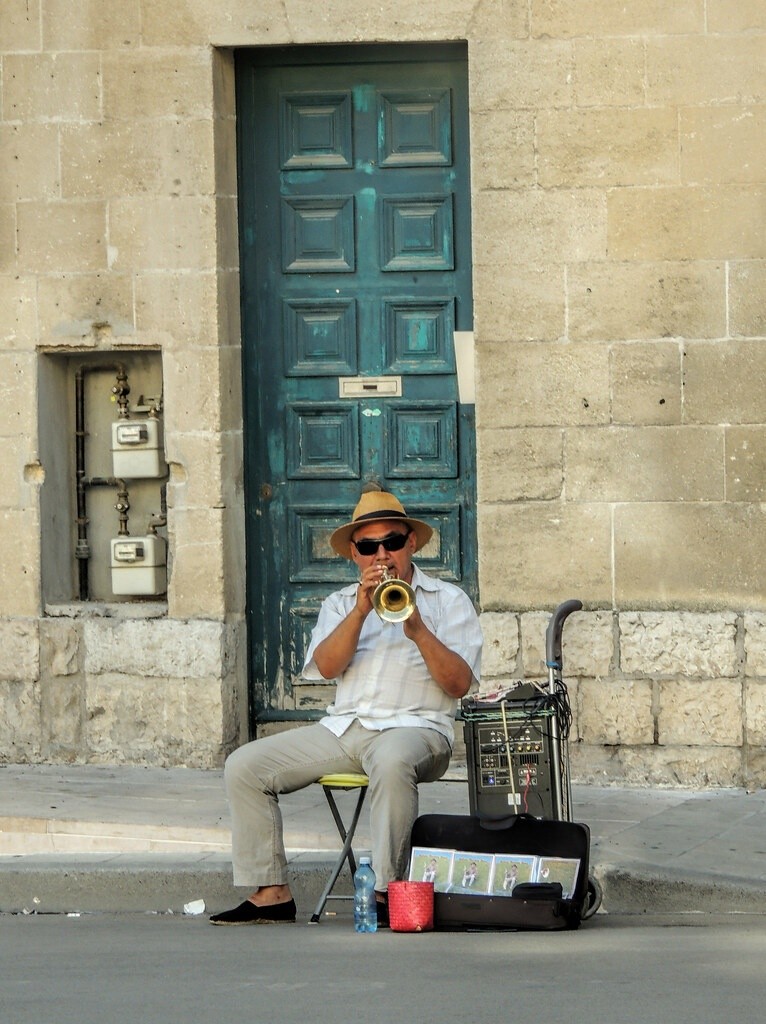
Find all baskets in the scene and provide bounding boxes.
[386,880,435,932]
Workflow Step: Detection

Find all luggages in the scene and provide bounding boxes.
[392,812,593,931]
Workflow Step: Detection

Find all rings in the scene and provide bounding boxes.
[359,582,363,587]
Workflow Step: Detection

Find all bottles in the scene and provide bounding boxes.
[354,857,377,933]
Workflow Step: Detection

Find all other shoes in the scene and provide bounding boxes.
[373,899,388,925]
[210,900,297,924]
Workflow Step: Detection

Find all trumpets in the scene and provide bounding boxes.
[371,568,418,624]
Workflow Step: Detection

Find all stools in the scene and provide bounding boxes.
[310,772,369,922]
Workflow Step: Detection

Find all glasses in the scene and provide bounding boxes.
[347,528,412,556]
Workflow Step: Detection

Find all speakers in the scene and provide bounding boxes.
[463,714,564,822]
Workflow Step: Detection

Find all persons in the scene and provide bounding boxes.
[207,491,484,927]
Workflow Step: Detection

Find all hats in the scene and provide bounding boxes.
[328,490,433,556]
